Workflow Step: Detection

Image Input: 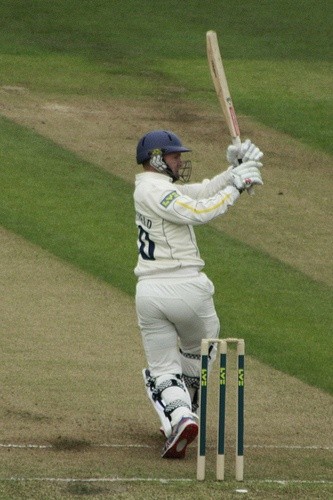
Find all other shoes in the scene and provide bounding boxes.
[161,415,199,459]
[159,425,168,437]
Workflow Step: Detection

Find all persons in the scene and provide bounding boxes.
[132,131,265,460]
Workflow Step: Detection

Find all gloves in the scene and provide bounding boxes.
[225,139,263,166]
[232,160,265,193]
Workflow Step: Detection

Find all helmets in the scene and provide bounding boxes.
[136,130,191,169]
